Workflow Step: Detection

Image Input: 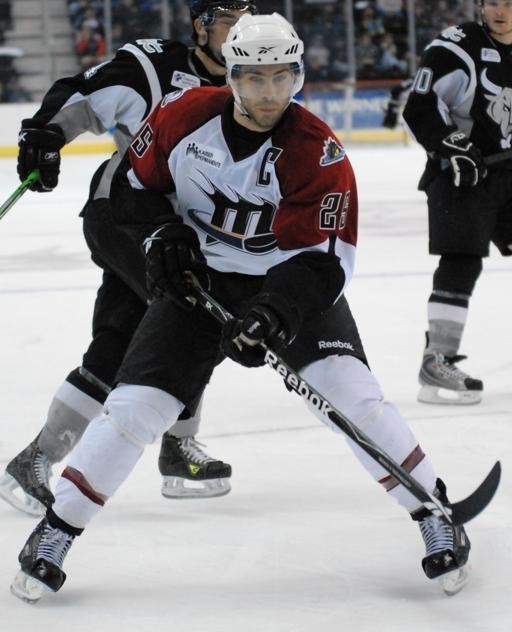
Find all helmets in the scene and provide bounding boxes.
[222,12,305,117]
[189,0,258,28]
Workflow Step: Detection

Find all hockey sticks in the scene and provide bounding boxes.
[184,270,500,526]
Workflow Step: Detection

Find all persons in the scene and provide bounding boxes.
[17,10,471,589]
[401,0,512,391]
[3,0,261,506]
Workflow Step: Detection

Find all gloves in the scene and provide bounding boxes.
[220,292,303,367]
[140,214,213,310]
[437,130,487,188]
[17,119,66,193]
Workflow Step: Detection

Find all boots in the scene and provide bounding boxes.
[410,479,470,578]
[158,432,231,479]
[418,331,484,391]
[7,430,54,505]
[19,506,84,591]
